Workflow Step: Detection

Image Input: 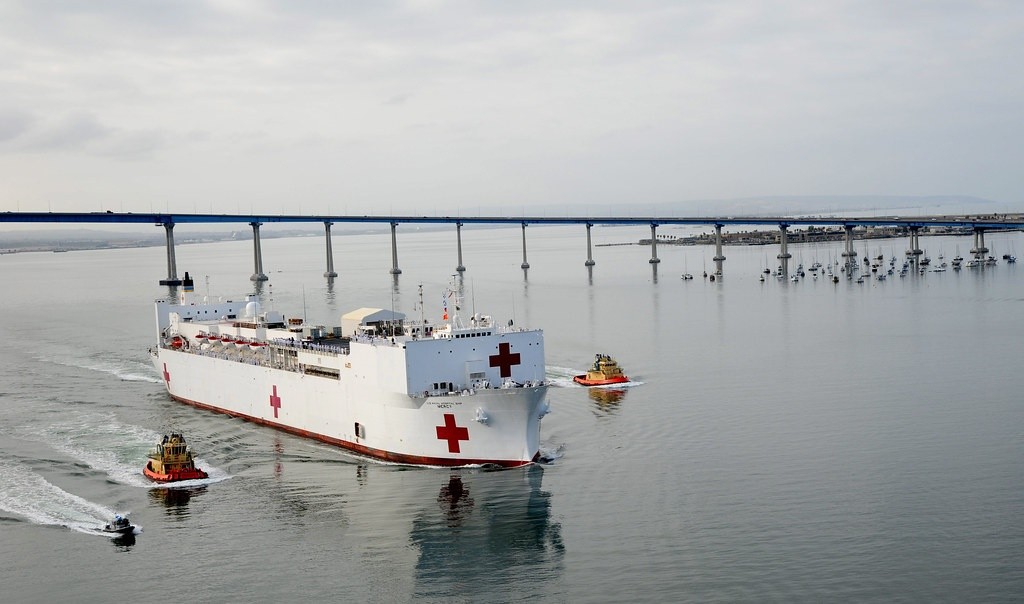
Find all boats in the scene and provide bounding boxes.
[142,439,208,484]
[147,284,553,469]
[573,352,631,387]
[102,521,136,535]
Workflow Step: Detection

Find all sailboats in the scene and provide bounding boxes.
[679,239,1018,283]
